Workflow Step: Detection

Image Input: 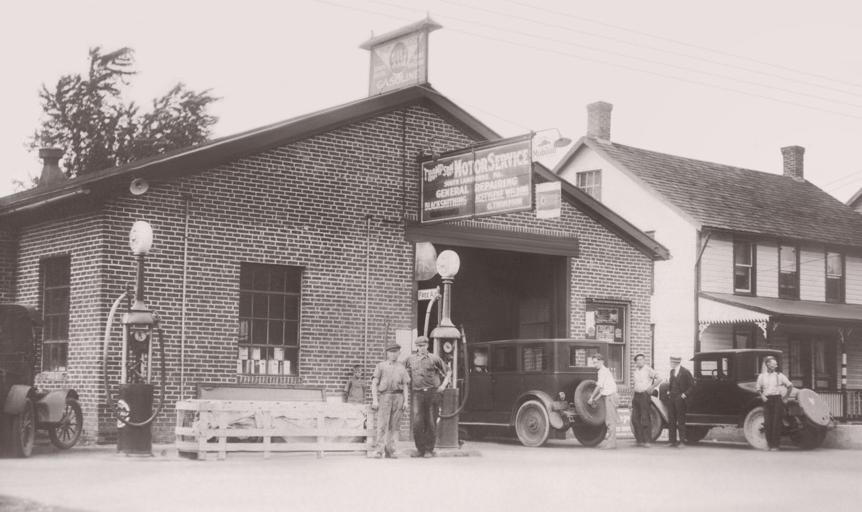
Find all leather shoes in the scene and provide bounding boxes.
[374,450,435,458]
[597,442,687,451]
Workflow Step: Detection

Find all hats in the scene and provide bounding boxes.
[414,335,430,347]
[669,356,682,364]
[633,352,645,361]
[385,343,401,352]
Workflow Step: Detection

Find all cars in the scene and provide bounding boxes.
[0,302,84,458]
[629,347,830,447]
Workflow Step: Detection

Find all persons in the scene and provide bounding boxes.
[631,354,662,447]
[372,343,411,459]
[400,336,452,457]
[663,355,694,448]
[587,354,618,450]
[755,356,793,452]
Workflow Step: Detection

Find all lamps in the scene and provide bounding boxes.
[533,126,571,149]
[129,174,149,196]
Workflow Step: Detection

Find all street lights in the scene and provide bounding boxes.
[118,217,157,455]
[432,247,463,446]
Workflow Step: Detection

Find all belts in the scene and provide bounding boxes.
[381,390,403,394]
[420,388,435,393]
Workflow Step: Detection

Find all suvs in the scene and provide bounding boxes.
[454,337,609,448]
